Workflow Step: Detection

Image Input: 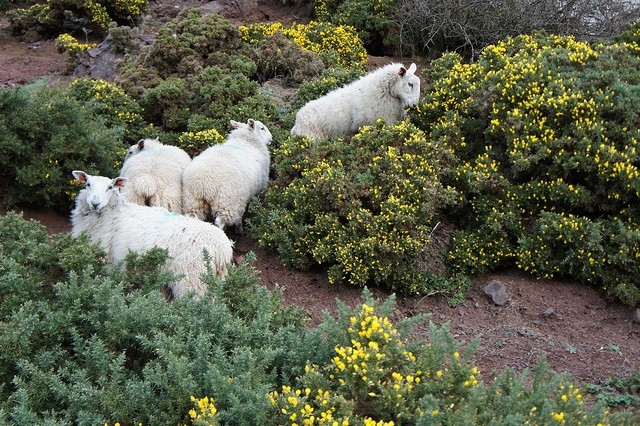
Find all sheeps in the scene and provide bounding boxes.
[181,118,275,233]
[289,60,423,143]
[118,136,194,215]
[69,168,237,304]
